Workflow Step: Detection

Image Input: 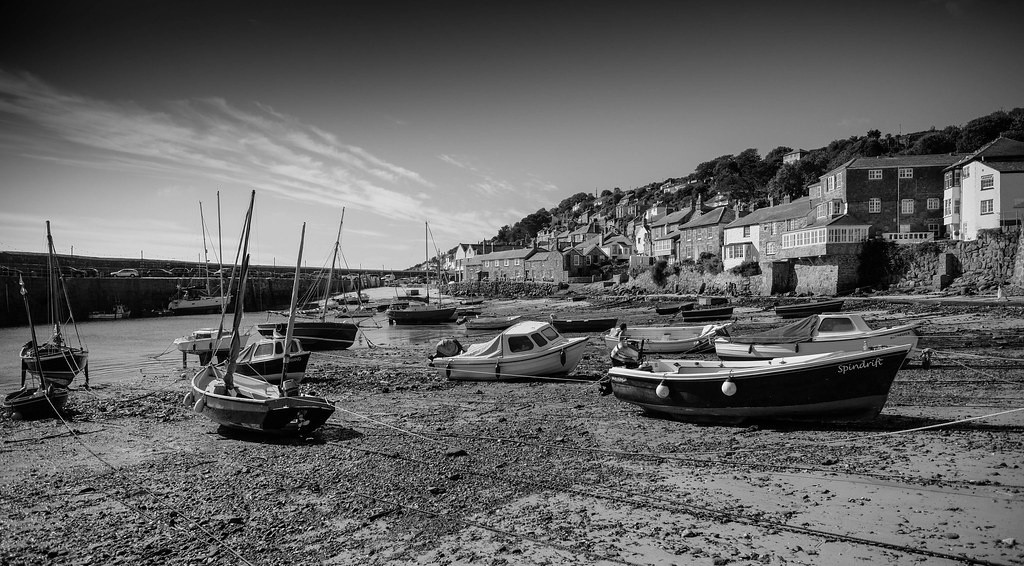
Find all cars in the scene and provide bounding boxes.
[0,266,24,276]
[110,269,140,278]
[171,267,431,284]
[146,269,173,276]
[80,268,98,277]
[58,265,88,278]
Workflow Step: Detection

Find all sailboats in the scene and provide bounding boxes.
[190,187,337,434]
[4,273,69,417]
[18,221,89,381]
[383,217,456,324]
[259,205,358,352]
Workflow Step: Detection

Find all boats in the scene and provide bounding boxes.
[680,305,738,320]
[715,312,929,360]
[232,330,312,383]
[775,297,845,318]
[456,309,482,316]
[601,316,739,354]
[465,314,522,332]
[88,302,131,319]
[426,321,592,383]
[165,279,235,313]
[654,300,695,315]
[605,340,913,426]
[460,294,485,306]
[549,313,619,331]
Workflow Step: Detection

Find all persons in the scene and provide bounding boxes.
[618,322,631,342]
